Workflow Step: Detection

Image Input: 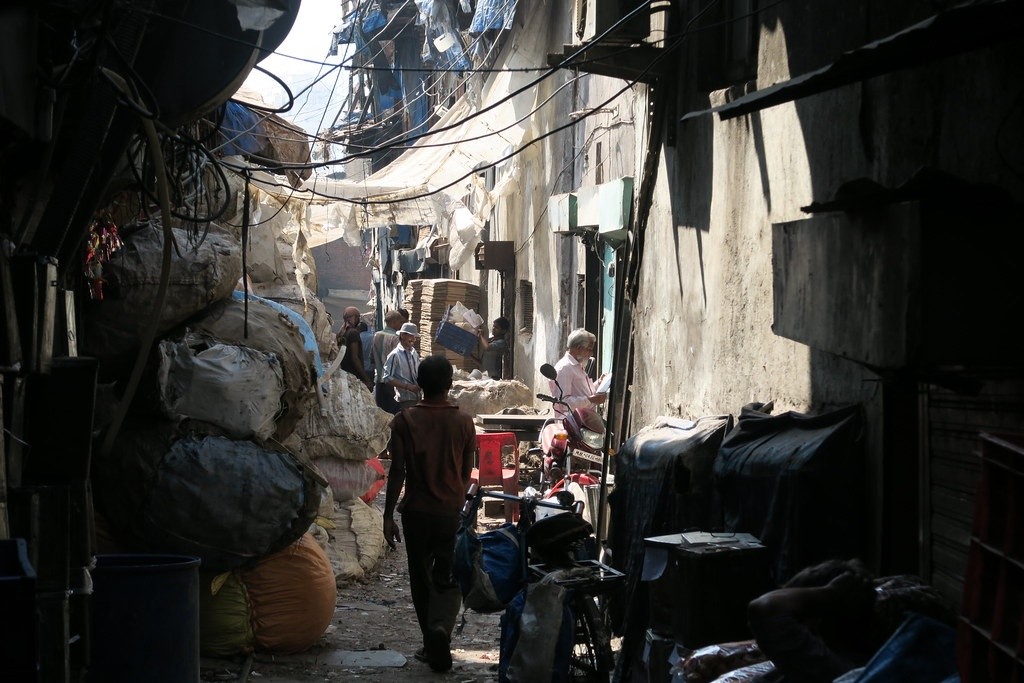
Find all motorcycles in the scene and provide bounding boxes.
[532,360,613,494]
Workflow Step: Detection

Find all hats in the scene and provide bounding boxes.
[395,322,422,338]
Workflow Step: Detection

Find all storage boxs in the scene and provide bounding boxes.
[434,304,480,359]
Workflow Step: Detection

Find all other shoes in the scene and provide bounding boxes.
[429,626,453,674]
[415,647,430,663]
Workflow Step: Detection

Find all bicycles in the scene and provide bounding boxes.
[446,483,626,683]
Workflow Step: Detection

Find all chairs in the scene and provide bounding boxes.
[471,432,521,524]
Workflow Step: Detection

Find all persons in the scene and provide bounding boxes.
[383,354,480,670]
[546,328,607,428]
[335,306,375,394]
[356,321,375,386]
[368,310,405,408]
[469,317,511,380]
[380,322,427,410]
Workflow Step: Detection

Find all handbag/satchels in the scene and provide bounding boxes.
[453,522,524,615]
[374,377,402,415]
